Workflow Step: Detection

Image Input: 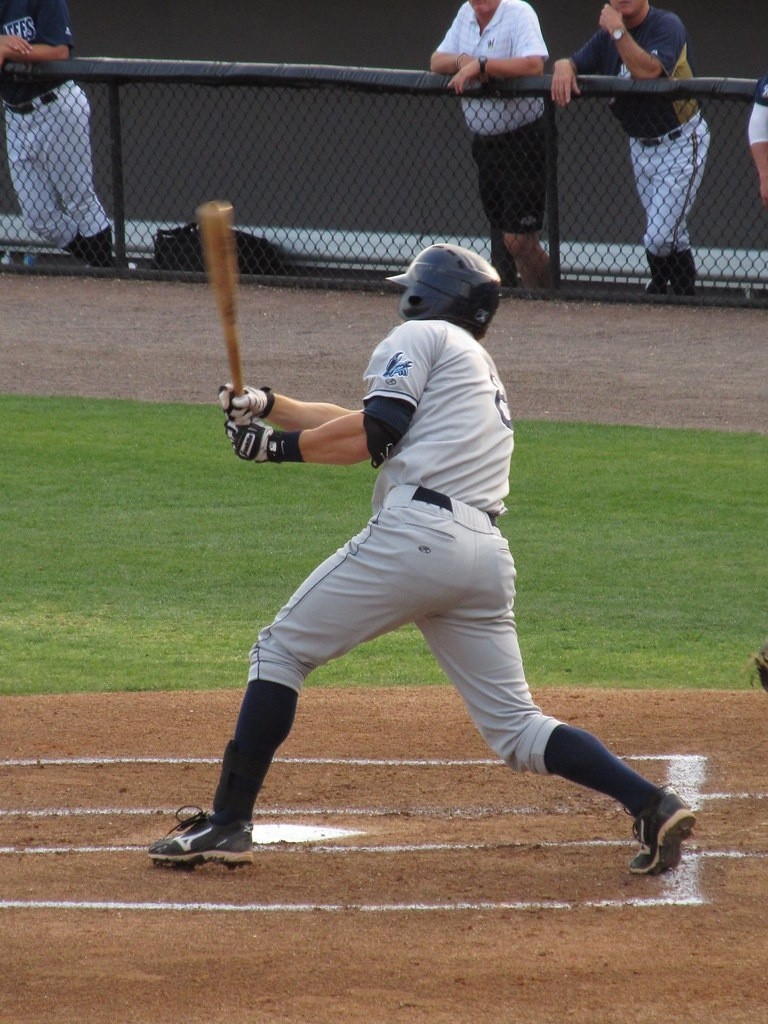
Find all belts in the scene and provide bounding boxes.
[636,119,702,146]
[412,486,499,527]
[5,93,56,115]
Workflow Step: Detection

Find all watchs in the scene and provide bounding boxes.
[610,25,627,41]
[478,55,488,74]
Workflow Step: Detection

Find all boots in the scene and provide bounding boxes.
[645,248,697,296]
[61,224,113,269]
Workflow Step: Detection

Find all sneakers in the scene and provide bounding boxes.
[628,795,696,874]
[148,806,253,868]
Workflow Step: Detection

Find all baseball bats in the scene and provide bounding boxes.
[195,200,242,396]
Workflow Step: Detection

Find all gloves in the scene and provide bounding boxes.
[219,382,274,421]
[224,420,280,463]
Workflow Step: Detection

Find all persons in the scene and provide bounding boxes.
[748,74,768,208]
[0,0,116,268]
[146,245,695,874]
[429,0,554,288]
[551,0,710,295]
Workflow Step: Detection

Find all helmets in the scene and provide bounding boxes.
[386,242,500,332]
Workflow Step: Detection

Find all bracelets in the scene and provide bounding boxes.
[455,52,467,70]
